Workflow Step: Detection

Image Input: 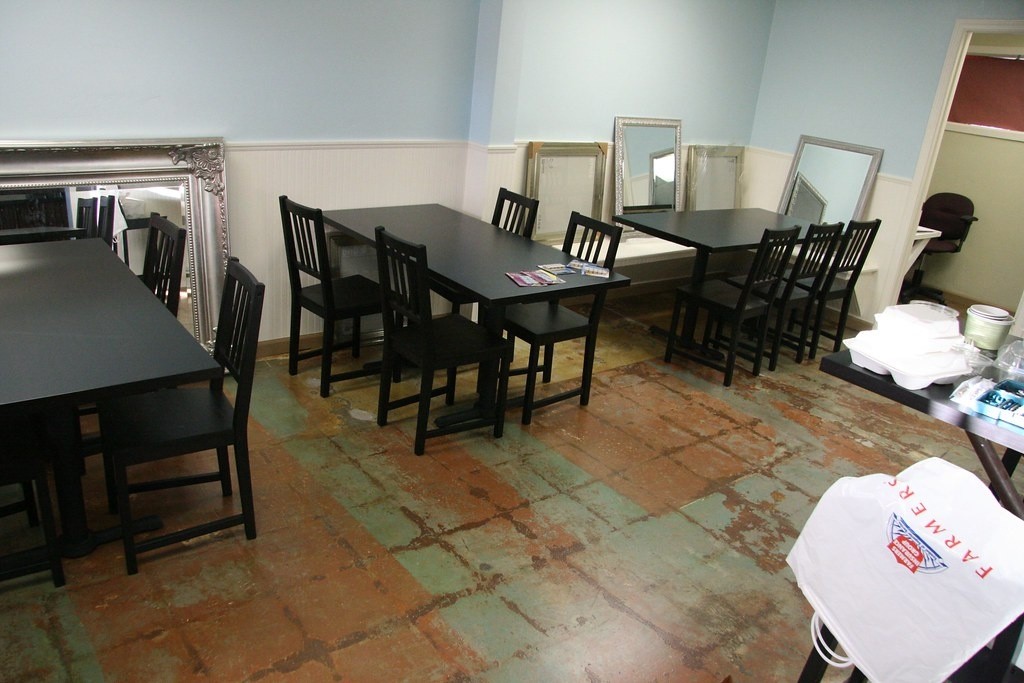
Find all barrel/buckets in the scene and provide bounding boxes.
[964,304,1016,350]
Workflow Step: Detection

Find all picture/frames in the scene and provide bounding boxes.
[684,144,744,211]
[524,141,609,246]
[323,231,407,348]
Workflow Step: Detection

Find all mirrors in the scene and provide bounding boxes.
[614,116,682,239]
[0,137,237,377]
[785,171,828,249]
[775,133,885,259]
[648,147,676,209]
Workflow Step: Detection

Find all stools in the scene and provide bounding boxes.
[794,611,1024,683]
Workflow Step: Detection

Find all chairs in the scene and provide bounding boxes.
[374,226,514,456]
[664,224,802,387]
[0,416,65,587]
[698,222,845,371]
[746,218,882,361]
[279,195,402,398]
[98,254,265,577]
[482,211,623,425]
[75,197,97,240]
[451,187,540,363]
[96,195,115,250]
[897,192,978,305]
[73,207,188,456]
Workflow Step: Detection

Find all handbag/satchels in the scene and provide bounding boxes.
[785,457,1024,683]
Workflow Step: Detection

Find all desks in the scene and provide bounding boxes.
[904,225,942,276]
[817,317,1024,520]
[0,226,87,246]
[611,207,854,362]
[322,204,632,429]
[0,237,223,575]
